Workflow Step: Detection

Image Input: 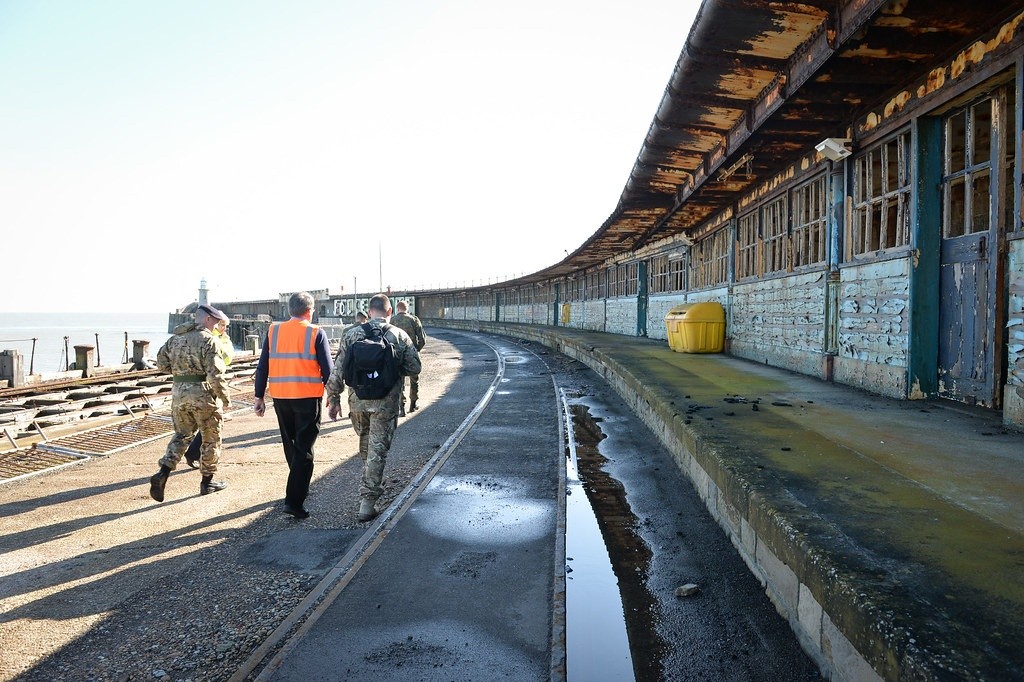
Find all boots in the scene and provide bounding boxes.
[357,490,384,521]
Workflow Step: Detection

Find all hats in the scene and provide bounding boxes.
[197,303,222,321]
[356,311,369,318]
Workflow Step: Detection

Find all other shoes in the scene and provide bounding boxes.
[150,465,171,502]
[399,405,405,417]
[409,402,418,411]
[185,456,199,467]
[282,503,310,518]
[200,475,228,496]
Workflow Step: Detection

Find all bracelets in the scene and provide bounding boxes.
[255,397,263,401]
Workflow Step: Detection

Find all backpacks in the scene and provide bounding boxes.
[342,322,400,399]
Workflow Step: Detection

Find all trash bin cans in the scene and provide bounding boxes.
[665,302,726,354]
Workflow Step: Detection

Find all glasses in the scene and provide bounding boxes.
[307,305,317,313]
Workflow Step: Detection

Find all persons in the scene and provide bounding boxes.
[326,293,427,522]
[253,291,334,519]
[150,303,235,502]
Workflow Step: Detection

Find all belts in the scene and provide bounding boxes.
[170,374,208,382]
